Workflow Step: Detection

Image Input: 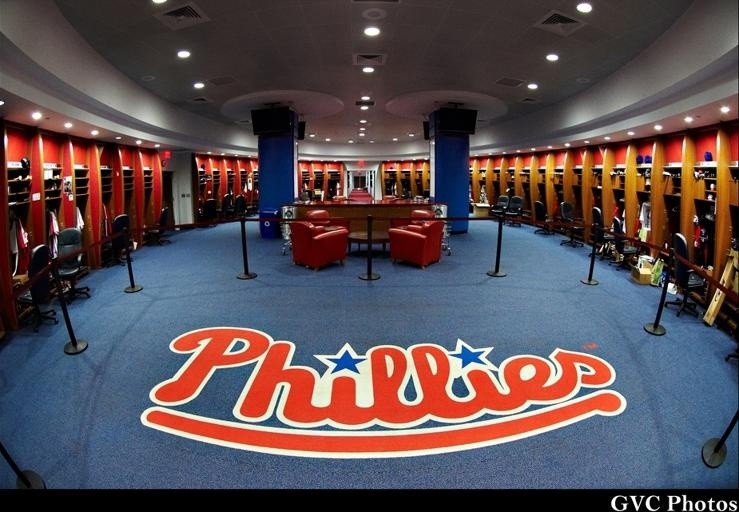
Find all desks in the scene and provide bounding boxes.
[348,231,390,252]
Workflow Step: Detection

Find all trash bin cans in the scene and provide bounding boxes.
[259,207,280,238]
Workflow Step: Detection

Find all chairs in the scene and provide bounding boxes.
[559,201,584,249]
[492,195,526,227]
[587,208,610,255]
[286,220,348,270]
[665,231,703,319]
[535,200,555,235]
[611,218,639,269]
[387,218,444,266]
[305,209,333,224]
[17,194,245,333]
[410,209,433,221]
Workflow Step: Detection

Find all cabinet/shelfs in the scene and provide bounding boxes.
[7,163,340,208]
[383,164,739,209]
